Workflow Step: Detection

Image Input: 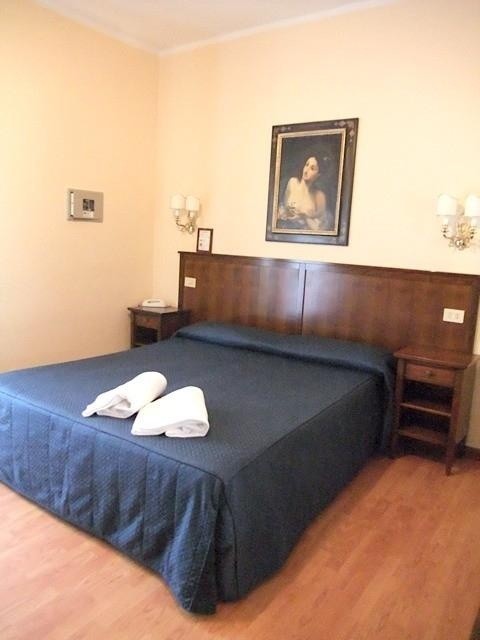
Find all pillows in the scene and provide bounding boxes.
[178,320,396,375]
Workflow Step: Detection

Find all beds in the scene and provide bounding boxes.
[0,335,393,615]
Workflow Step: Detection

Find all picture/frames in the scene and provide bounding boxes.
[265,117,359,246]
[196,228,213,253]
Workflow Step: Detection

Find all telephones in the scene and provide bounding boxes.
[142,299,167,307]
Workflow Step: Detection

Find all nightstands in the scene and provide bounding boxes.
[126,305,191,349]
[391,345,480,475]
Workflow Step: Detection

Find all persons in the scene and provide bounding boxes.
[275,149,329,231]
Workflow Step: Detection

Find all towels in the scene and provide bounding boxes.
[130,384,210,439]
[81,371,168,419]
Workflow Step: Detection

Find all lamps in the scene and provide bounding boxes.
[169,195,199,234]
[436,191,480,250]
